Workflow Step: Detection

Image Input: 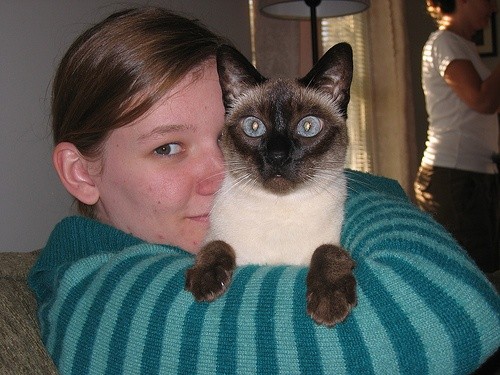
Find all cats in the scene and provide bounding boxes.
[182,40,361,330]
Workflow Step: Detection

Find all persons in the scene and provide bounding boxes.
[25,5,500,375]
[414,1,500,273]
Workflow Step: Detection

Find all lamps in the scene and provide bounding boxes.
[259,0,370,67]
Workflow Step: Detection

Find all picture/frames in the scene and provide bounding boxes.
[471,12,498,57]
